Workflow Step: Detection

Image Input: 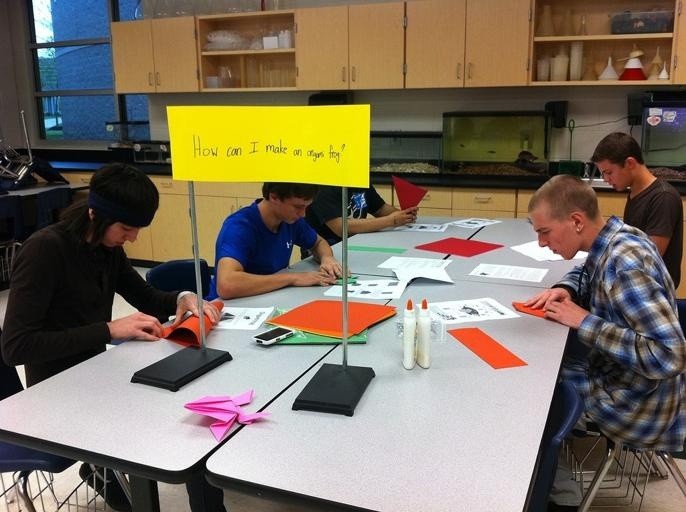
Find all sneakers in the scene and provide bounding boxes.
[80,462,130,512]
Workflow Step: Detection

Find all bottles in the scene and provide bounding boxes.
[536,4,586,36]
[402,298,432,369]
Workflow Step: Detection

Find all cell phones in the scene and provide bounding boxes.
[251,326,295,346]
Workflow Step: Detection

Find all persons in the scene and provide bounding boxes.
[301,180,419,260]
[590,132,683,290]
[528,173,685,455]
[1,164,226,511]
[207,183,352,301]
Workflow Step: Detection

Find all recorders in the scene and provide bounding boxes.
[610,10,669,33]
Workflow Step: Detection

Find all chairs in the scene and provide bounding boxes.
[0,109,70,190]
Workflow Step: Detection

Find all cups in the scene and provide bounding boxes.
[218,66,234,88]
[538,42,584,81]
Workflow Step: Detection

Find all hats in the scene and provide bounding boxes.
[87,163,159,227]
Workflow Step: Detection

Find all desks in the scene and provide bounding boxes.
[0,183,90,293]
[0,216,589,512]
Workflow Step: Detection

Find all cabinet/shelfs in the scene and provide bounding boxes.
[196,9,296,92]
[673,0,686,85]
[296,1,404,91]
[59,172,686,299]
[405,0,531,89]
[528,0,680,86]
[111,16,200,94]
